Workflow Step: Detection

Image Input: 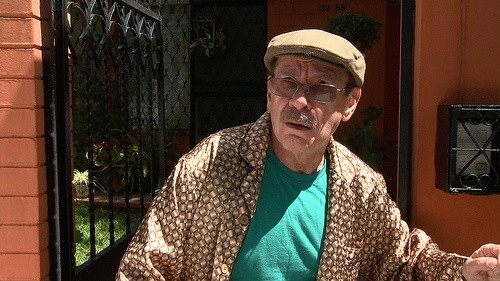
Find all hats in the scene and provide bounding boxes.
[264,29,366,88]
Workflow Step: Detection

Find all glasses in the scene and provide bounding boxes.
[267,75,348,103]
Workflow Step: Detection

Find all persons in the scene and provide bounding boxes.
[114,28,500,281]
